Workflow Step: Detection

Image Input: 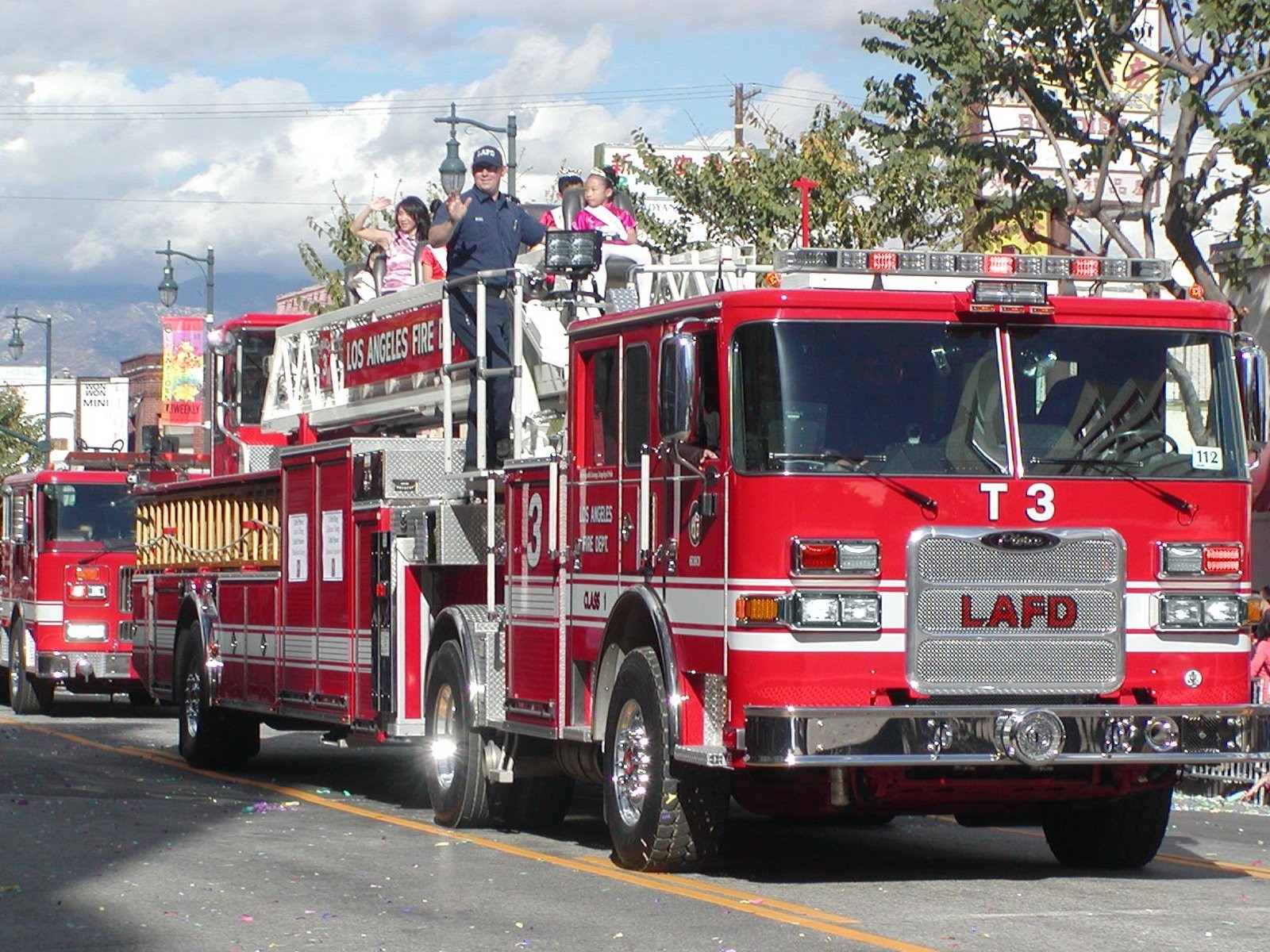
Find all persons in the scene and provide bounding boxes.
[1252,590,1260,600]
[1250,609,1270,704]
[1260,585,1270,619]
[429,146,561,478]
[348,245,385,322]
[528,175,584,291]
[572,164,652,321]
[349,196,431,295]
[45,499,97,542]
[421,199,447,284]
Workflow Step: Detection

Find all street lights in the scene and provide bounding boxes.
[153,237,215,456]
[434,102,518,202]
[4,305,53,467]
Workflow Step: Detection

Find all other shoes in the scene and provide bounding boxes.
[463,462,481,472]
[488,457,503,470]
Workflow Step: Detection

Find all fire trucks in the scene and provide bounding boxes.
[127,180,1270,870]
[0,438,214,717]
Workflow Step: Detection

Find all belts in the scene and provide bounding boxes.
[457,282,507,299]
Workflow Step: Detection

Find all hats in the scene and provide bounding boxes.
[473,146,502,168]
